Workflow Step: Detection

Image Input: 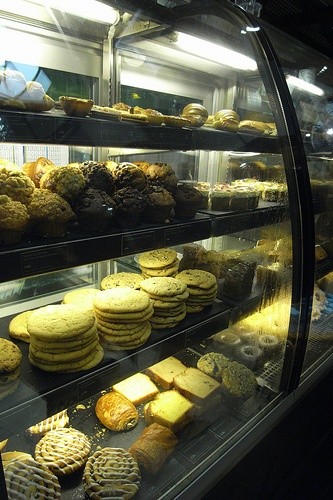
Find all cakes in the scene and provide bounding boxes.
[194,178,333,210]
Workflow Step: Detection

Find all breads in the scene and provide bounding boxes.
[0,352,258,500]
[245,271,333,336]
[0,68,277,136]
[0,156,206,248]
[178,212,333,298]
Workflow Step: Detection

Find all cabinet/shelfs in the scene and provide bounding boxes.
[0,0,333,500]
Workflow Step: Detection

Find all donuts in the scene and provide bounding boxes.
[212,325,280,371]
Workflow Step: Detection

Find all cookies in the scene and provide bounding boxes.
[0,248,219,384]
